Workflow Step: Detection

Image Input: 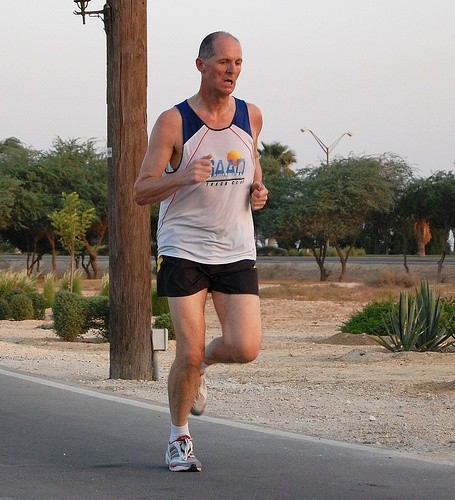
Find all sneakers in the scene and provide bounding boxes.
[165,434,202,472]
[190,366,207,415]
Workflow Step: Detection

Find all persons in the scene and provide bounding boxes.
[133,30,269,472]
